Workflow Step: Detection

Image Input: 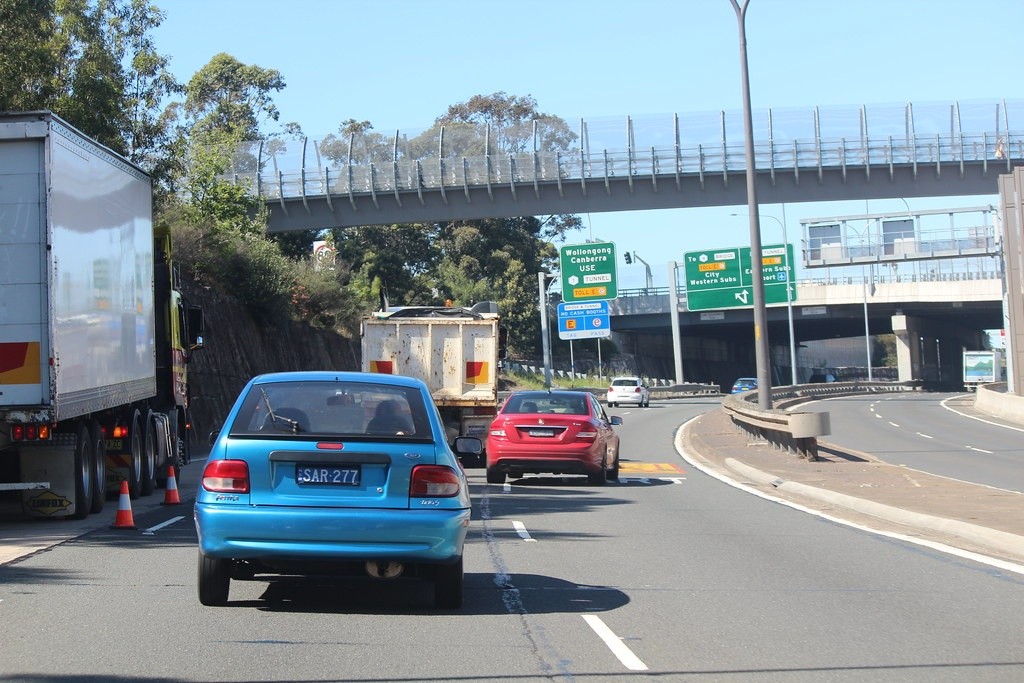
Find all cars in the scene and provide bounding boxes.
[732,377,757,394]
[606,377,650,407]
[809,374,836,383]
[195,371,483,608]
[485,390,623,484]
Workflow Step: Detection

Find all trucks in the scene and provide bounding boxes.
[962,350,1002,392]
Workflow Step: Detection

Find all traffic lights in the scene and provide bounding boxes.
[624,251,632,264]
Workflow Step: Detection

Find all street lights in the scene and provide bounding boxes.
[730,213,797,385]
[838,217,885,381]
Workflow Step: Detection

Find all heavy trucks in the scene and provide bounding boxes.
[360,301,507,467]
[0,111,204,519]
